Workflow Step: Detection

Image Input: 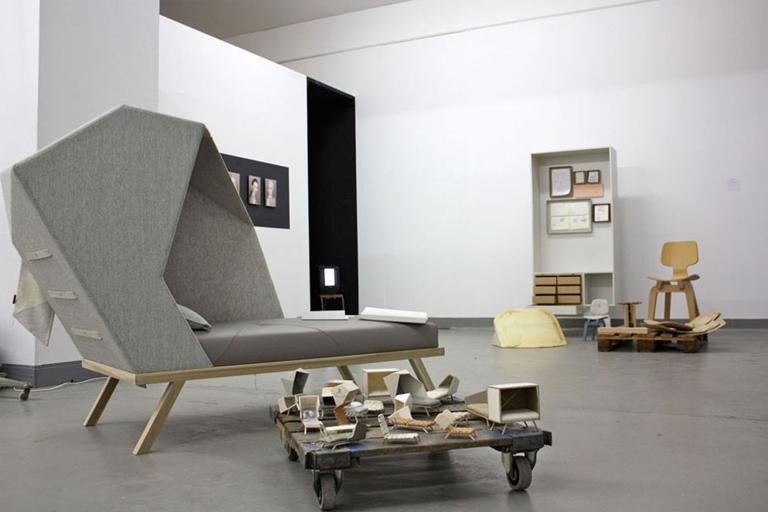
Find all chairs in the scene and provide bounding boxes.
[580,298,612,342]
[646,241,702,323]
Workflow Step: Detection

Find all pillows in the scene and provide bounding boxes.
[175,303,212,332]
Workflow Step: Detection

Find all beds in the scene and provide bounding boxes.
[276,367,542,451]
[1,100,447,457]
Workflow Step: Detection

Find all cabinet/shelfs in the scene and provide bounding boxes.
[529,144,620,320]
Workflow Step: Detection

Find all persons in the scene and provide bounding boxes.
[266,181,275,206]
[249,179,259,205]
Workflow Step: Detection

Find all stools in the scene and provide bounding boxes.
[616,299,643,327]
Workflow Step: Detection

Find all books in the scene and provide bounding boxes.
[300,310,346,320]
[359,306,429,325]
